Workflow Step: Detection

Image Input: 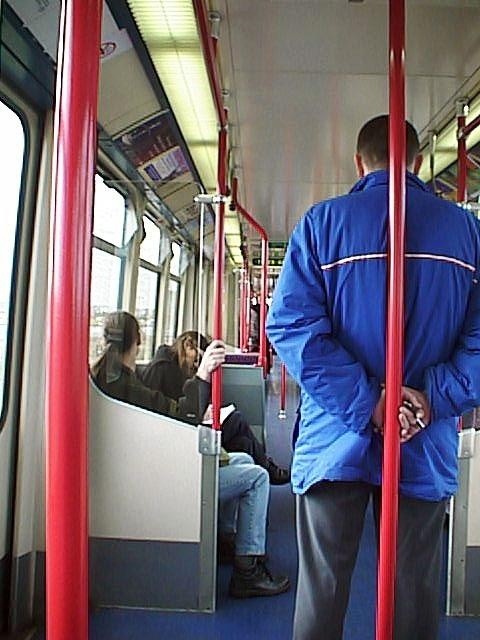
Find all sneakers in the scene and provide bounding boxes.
[259,456,290,485]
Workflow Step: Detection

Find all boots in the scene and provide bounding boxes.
[218,533,267,564]
[228,558,290,597]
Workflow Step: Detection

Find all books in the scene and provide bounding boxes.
[200,400,236,431]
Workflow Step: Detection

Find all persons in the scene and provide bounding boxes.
[263,111,480,638]
[92,307,291,600]
[251,293,270,360]
[145,326,293,490]
